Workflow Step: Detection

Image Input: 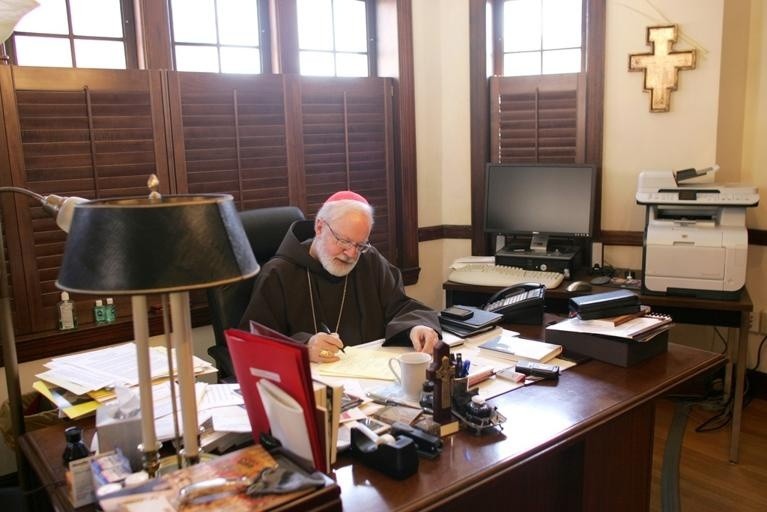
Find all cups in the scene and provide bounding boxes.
[389,352,433,400]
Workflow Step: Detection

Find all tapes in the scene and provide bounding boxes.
[354,423,397,445]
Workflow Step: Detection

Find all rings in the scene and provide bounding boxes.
[320,350,331,357]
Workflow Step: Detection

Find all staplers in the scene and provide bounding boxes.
[391,423,443,460]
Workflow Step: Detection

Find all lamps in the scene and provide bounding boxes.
[0,186,93,233]
[54,172,261,480]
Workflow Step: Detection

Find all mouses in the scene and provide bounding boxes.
[566,280,592,292]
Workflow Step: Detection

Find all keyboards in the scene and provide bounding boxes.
[447,264,564,290]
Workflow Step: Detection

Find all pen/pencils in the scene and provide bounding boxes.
[450,354,470,378]
[320,321,347,354]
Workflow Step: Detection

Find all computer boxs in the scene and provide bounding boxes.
[495,243,582,281]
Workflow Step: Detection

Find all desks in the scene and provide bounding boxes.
[15,316,730,510]
[442,268,752,460]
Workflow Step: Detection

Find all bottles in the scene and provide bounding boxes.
[63,428,89,496]
[94,299,115,323]
[59,293,74,330]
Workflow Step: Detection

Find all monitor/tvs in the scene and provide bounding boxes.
[483,162,597,254]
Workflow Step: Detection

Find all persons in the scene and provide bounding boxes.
[546,289,676,341]
[237,191,442,364]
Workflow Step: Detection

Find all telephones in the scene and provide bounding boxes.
[484,282,544,325]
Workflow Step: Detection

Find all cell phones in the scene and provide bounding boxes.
[440,305,474,321]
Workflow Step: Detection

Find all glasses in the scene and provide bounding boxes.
[319,218,371,254]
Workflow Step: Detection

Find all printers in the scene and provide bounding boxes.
[634,164,761,302]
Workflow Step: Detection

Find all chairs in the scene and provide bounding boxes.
[207,204,307,383]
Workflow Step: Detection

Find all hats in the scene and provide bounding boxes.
[325,191,370,205]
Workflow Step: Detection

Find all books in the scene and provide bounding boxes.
[33,321,345,512]
[439,304,503,339]
[449,256,495,270]
[478,334,563,363]
[467,363,494,388]
